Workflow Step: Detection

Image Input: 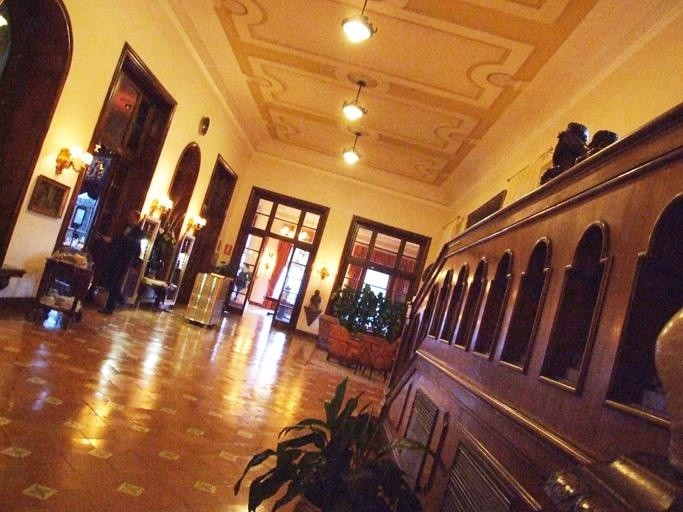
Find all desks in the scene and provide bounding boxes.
[30,256,96,328]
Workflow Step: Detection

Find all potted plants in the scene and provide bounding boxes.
[231,378,447,512]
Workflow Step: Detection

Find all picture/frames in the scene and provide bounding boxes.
[27,174,71,219]
[119,213,196,305]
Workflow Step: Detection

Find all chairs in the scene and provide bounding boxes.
[309,315,401,383]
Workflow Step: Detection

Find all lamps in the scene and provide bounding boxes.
[339,80,372,164]
[57,147,93,174]
[340,0,379,45]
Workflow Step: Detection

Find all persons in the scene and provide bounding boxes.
[84,202,143,315]
